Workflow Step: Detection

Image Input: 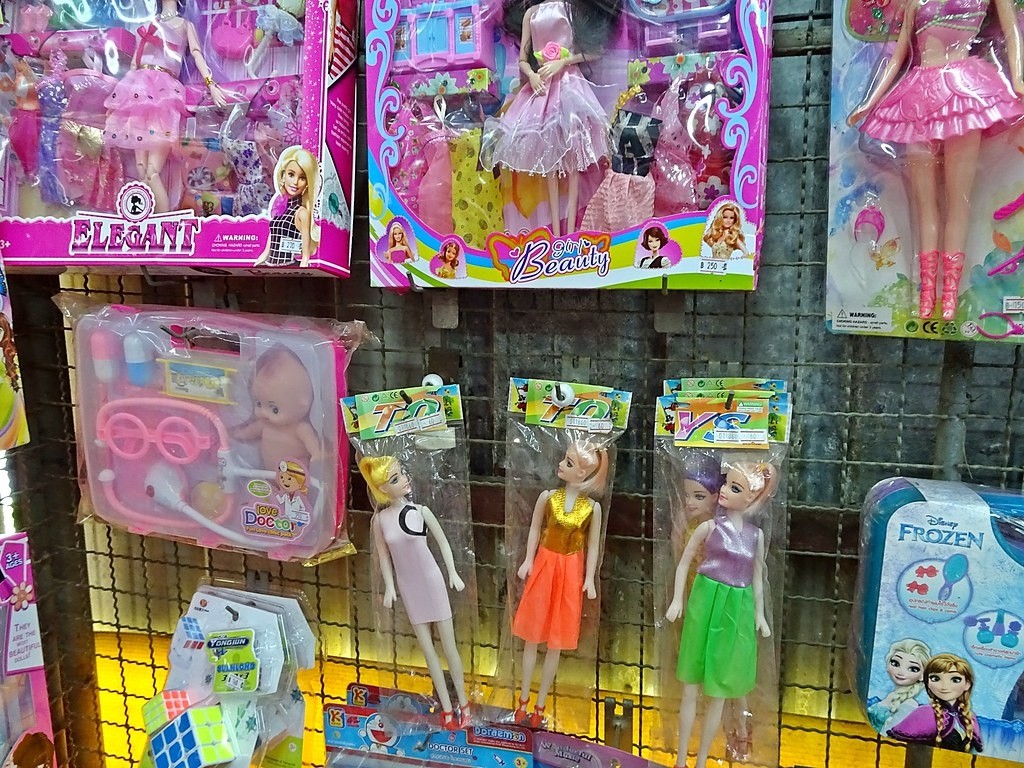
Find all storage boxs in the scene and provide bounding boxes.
[846,476,1024,763]
[0,0,359,278]
[364,0,773,291]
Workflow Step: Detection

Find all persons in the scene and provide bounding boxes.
[0,0,1020,768]
